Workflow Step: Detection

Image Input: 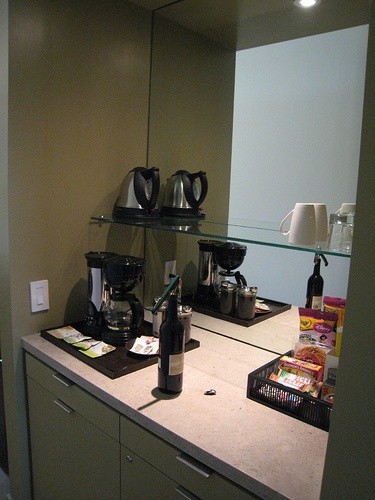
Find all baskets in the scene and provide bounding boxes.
[245,350,333,433]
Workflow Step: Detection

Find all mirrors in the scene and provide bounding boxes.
[142,0,373,356]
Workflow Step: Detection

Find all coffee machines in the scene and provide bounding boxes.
[82,251,144,345]
[193,240,248,306]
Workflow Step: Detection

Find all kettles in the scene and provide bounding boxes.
[163,170,208,219]
[116,165,162,219]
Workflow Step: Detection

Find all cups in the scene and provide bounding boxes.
[152,297,168,336]
[280,202,317,245]
[335,202,356,214]
[235,287,258,320]
[176,304,193,345]
[312,203,328,243]
[218,284,237,313]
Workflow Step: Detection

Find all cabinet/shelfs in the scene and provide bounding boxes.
[122,416,261,500]
[25,353,120,500]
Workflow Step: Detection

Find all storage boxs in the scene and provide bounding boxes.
[244,348,332,434]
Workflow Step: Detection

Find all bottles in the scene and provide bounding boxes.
[158,274,185,395]
[305,254,323,312]
[326,213,355,252]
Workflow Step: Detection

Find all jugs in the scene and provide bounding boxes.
[213,271,246,296]
[103,291,144,333]
